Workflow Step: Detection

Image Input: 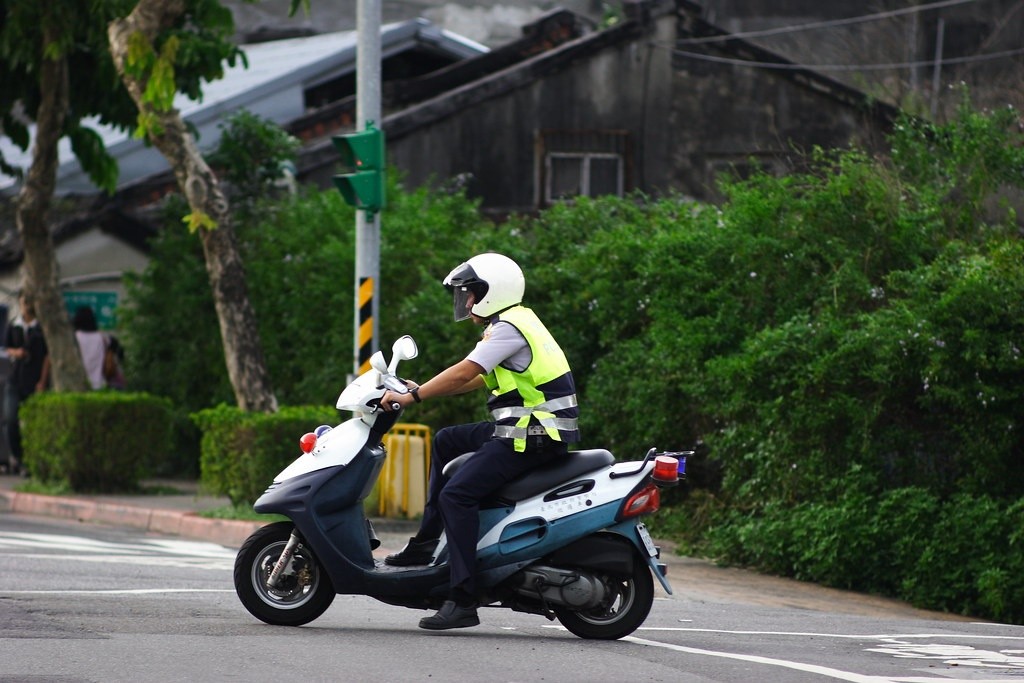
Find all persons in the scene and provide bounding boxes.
[380,254,578,630]
[0,288,126,475]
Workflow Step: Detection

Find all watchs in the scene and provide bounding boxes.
[409,387,421,402]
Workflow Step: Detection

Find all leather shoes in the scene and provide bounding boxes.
[382,544,432,567]
[418,599,481,630]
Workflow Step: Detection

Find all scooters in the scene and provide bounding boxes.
[233,335,696,641]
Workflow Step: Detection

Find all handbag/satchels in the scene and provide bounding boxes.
[101,331,119,380]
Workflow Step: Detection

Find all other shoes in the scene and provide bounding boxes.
[9,456,23,474]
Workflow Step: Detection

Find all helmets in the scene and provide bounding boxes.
[443,252,525,320]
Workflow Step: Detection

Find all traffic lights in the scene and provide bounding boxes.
[328,120,391,214]
